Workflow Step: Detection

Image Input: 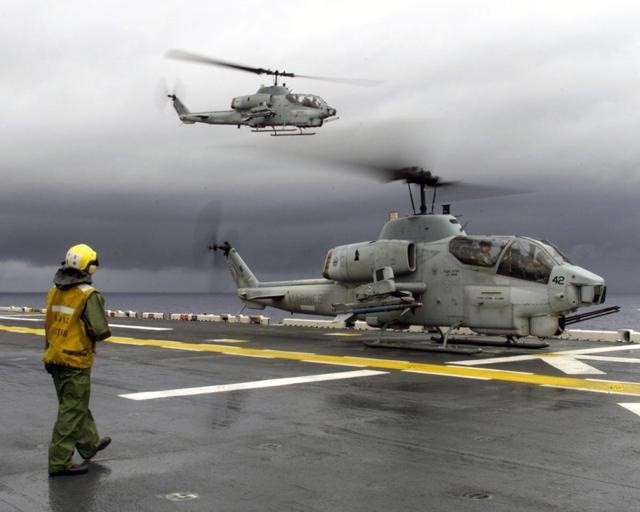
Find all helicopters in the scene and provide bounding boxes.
[210,165,620,355]
[167,51,382,137]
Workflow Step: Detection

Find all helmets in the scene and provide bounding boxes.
[64,243,96,270]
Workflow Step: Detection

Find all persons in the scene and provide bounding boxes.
[475,238,494,266]
[41,242,113,478]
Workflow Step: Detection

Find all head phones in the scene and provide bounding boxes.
[86,248,97,274]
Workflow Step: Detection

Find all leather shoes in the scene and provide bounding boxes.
[82,436,112,460]
[48,464,89,476]
